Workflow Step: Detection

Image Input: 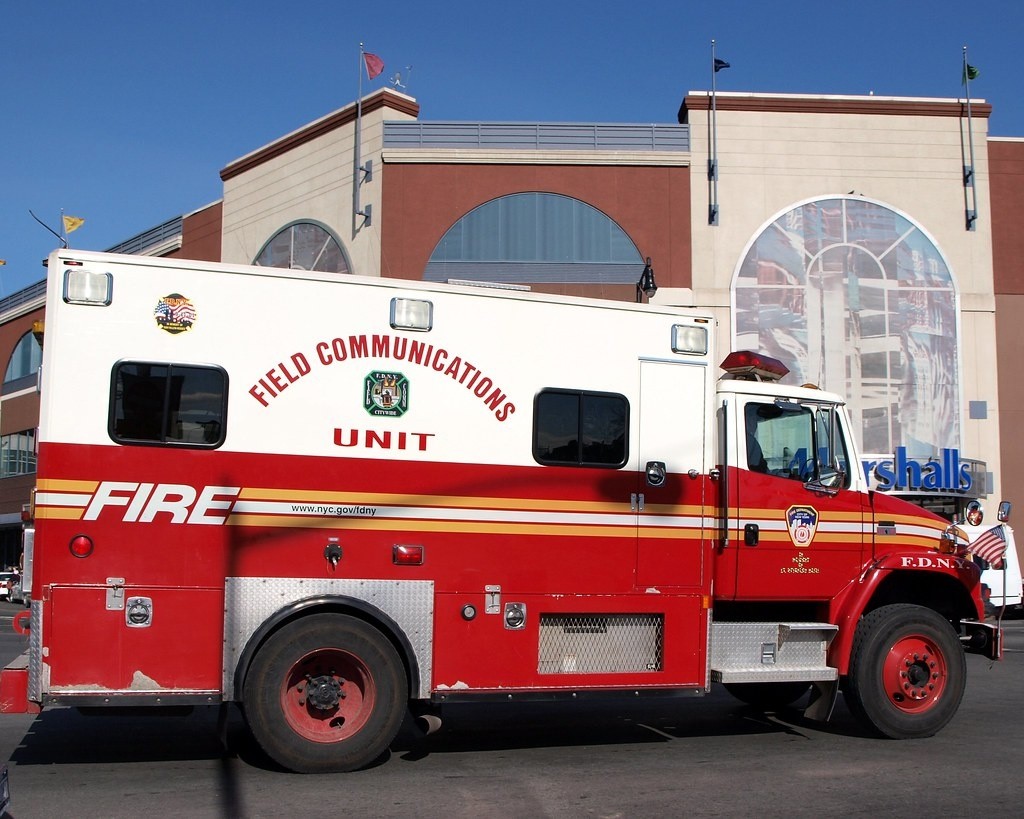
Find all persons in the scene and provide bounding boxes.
[968,504,979,525]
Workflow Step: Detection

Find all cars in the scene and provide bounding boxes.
[0,572,31,608]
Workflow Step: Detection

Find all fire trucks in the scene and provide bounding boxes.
[19,248,1014,777]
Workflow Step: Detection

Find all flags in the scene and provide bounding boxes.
[713,58,730,72]
[961,61,980,85]
[362,51,385,80]
[967,525,1006,566]
[63,215,86,234]
